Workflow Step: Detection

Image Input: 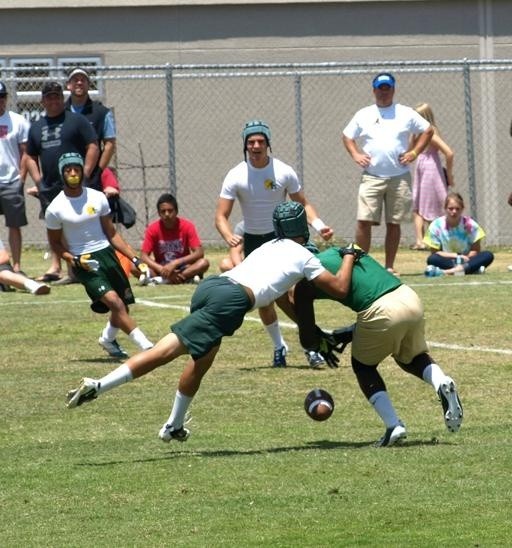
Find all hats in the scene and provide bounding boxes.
[68,67,91,80]
[0,81,8,95]
[240,119,274,164]
[56,151,86,190]
[271,200,311,244]
[41,80,64,98]
[371,72,396,90]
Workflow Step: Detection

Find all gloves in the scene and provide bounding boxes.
[337,240,364,263]
[317,332,343,372]
[326,324,353,354]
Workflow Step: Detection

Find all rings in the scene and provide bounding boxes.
[404,158,408,162]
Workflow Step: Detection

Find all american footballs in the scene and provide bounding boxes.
[304,390,334,420]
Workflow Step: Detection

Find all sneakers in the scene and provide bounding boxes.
[134,272,202,288]
[373,419,409,449]
[131,333,154,351]
[32,284,52,297]
[303,349,328,371]
[435,377,465,436]
[424,265,445,277]
[474,265,486,275]
[63,375,102,412]
[270,335,288,370]
[97,333,129,360]
[157,409,194,445]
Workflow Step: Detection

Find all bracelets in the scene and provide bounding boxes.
[310,218,326,234]
[409,149,419,161]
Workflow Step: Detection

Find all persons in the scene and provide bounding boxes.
[506,114,512,276]
[215,120,333,369]
[45,153,154,361]
[129,193,210,285]
[217,221,245,273]
[407,102,455,251]
[293,237,464,448]
[65,200,364,444]
[423,190,493,276]
[341,72,433,279]
[0,65,136,295]
